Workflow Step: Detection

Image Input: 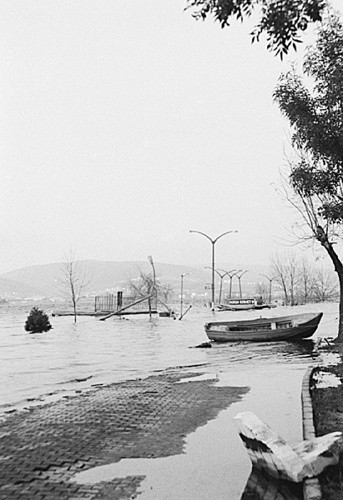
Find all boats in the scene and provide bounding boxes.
[203,312,323,342]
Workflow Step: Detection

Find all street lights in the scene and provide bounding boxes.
[189,230,280,304]
